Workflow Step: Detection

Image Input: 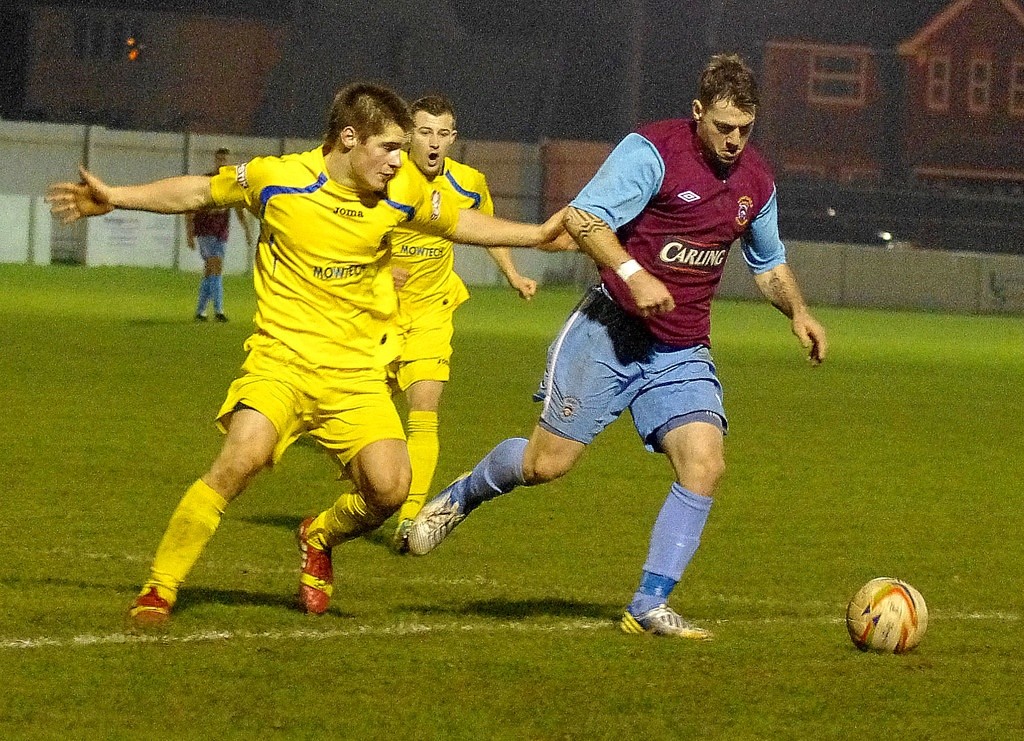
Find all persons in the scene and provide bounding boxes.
[184,147,252,322]
[408,54,828,638]
[43,81,571,625]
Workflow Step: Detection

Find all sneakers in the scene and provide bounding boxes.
[129,583,173,632]
[620,603,714,643]
[408,471,472,557]
[296,518,334,614]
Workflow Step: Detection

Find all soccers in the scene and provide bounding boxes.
[846,576,929,654]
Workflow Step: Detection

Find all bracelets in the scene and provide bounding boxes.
[617,259,643,282]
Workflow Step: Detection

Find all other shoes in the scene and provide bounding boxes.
[213,313,229,323]
[194,310,210,322]
[394,516,415,554]
